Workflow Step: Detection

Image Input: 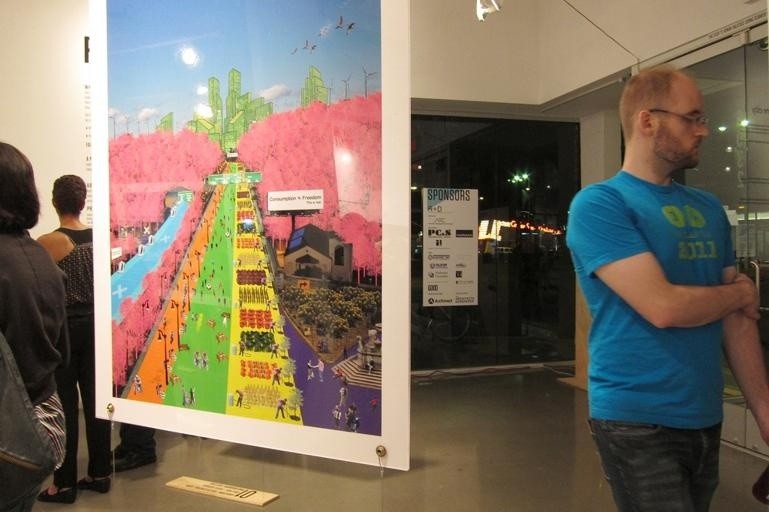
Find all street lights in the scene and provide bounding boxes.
[170,299,180,350]
[214,199,217,216]
[189,216,197,243]
[543,184,553,225]
[174,247,182,275]
[141,298,151,350]
[182,271,191,311]
[126,329,135,382]
[193,249,200,278]
[203,217,209,243]
[155,327,169,385]
[159,271,168,311]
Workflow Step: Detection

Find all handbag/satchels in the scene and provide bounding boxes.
[0,333,55,508]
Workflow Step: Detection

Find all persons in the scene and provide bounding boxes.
[564,66,769,512]
[31,171,112,504]
[0,140,70,512]
[105,422,159,474]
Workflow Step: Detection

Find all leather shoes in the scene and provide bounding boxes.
[38,484,76,503]
[111,445,127,457]
[79,476,110,493]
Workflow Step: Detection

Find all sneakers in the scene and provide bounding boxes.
[111,447,157,471]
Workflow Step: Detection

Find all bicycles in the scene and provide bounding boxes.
[411,298,471,343]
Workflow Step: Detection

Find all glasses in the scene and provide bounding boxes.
[647,108,708,125]
